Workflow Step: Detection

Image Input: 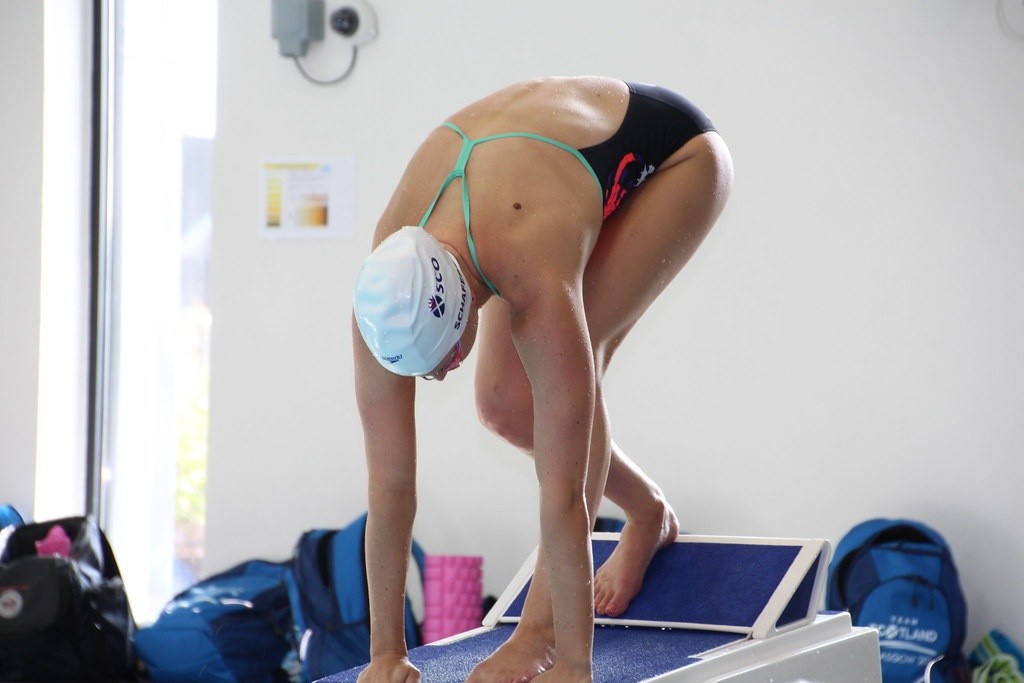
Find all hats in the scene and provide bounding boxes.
[354,226,471,377]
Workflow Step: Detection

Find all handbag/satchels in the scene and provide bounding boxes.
[282,513,426,680]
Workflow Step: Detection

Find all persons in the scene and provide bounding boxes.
[347,76,736,682]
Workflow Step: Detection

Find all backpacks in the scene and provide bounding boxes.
[825,518,967,683]
[0,515,139,683]
[132,560,297,683]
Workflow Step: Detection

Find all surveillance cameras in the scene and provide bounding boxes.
[331,0,377,45]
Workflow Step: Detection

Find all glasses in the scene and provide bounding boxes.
[421,341,464,381]
